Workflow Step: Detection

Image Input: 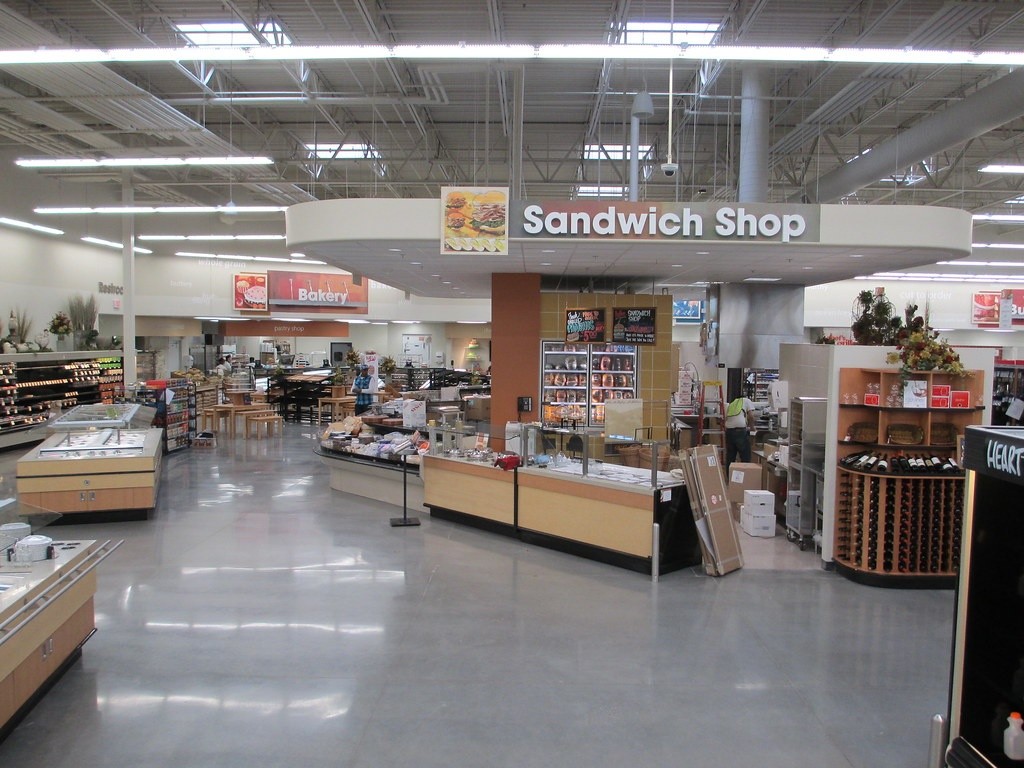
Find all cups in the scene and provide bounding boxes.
[842,379,903,408]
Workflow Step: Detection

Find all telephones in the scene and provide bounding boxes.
[518,397,531,412]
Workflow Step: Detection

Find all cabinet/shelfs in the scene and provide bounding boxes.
[785,396,826,556]
[741,368,780,403]
[832,367,985,589]
[392,367,446,393]
[135,383,197,456]
[267,378,333,424]
[541,341,638,427]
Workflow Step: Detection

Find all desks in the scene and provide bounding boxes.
[201,403,284,441]
[319,396,356,426]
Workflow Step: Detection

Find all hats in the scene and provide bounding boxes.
[360,364,369,371]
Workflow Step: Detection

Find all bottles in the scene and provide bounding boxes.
[837,450,964,574]
[1003,712,1024,760]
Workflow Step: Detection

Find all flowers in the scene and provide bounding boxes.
[344,349,360,366]
[49,311,73,334]
[886,324,976,397]
[380,356,397,375]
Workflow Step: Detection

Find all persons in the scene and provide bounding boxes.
[225,355,232,371]
[725,398,756,476]
[352,365,375,416]
[216,358,227,373]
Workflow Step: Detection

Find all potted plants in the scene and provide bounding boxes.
[331,364,347,399]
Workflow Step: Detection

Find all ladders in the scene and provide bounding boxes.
[698,381,725,464]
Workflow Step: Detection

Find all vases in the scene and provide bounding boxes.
[56,334,66,352]
[350,364,355,371]
[385,373,392,385]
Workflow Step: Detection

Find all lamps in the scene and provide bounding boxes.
[226,7,236,207]
[632,61,654,119]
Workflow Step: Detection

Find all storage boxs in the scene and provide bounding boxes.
[903,380,971,409]
[864,393,879,407]
[727,462,776,538]
[465,396,490,421]
[674,371,694,406]
[362,398,426,427]
